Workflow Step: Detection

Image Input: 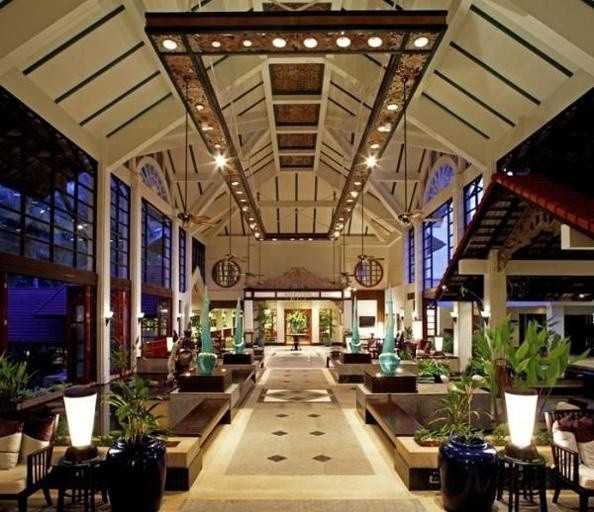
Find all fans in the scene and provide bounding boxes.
[162,72,267,287]
[321,76,439,292]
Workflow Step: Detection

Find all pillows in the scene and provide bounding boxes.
[550,411,582,464]
[571,411,594,470]
[0,419,26,471]
[13,408,57,465]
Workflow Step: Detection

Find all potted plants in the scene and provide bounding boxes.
[100,328,178,512]
[419,357,457,385]
[416,352,504,512]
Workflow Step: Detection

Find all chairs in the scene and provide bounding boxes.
[1,409,60,512]
[546,406,594,512]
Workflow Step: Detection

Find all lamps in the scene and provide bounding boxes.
[502,386,540,462]
[104,311,113,326]
[138,312,145,323]
[60,383,100,466]
[145,8,448,242]
[449,311,457,324]
[432,336,445,357]
[480,310,489,326]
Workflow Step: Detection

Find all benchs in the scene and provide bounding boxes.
[365,398,427,442]
[173,398,230,446]
[229,366,255,409]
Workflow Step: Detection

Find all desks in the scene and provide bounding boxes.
[285,333,308,351]
[52,451,110,512]
[492,450,549,512]
